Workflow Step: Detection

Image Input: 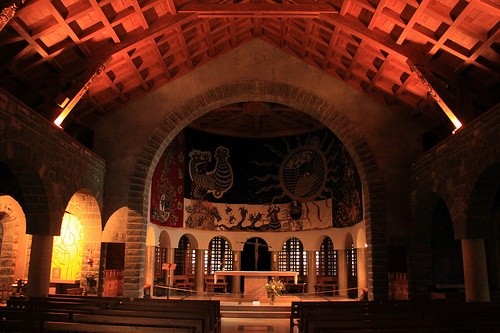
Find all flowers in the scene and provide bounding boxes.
[263,278,287,302]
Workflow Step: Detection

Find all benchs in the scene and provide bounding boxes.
[289,300,500,333]
[170,273,228,295]
[0,294,223,333]
[283,274,307,294]
[313,277,339,296]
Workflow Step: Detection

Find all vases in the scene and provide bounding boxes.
[270,294,276,306]
